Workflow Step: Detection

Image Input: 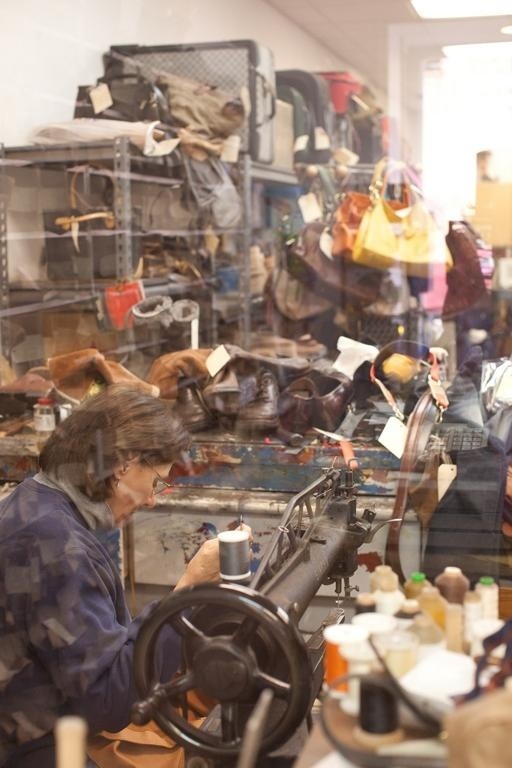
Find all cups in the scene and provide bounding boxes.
[322,612,391,714]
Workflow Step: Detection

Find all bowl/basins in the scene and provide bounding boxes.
[390,643,478,716]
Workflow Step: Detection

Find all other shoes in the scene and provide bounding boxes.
[50,297,354,433]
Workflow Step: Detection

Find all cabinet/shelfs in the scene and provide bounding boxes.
[0,137,303,401]
[306,157,388,344]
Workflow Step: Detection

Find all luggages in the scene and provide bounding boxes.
[274,70,390,164]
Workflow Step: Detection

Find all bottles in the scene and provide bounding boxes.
[31,397,57,439]
[357,559,502,655]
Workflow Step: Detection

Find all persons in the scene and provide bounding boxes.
[467,148,512,256]
[1,380,255,767]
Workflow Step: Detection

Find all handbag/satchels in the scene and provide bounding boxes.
[42,205,144,282]
[263,155,495,349]
[208,184,243,229]
[74,73,176,124]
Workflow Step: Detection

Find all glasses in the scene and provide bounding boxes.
[145,459,175,496]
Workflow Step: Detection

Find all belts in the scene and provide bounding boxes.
[384,346,452,568]
